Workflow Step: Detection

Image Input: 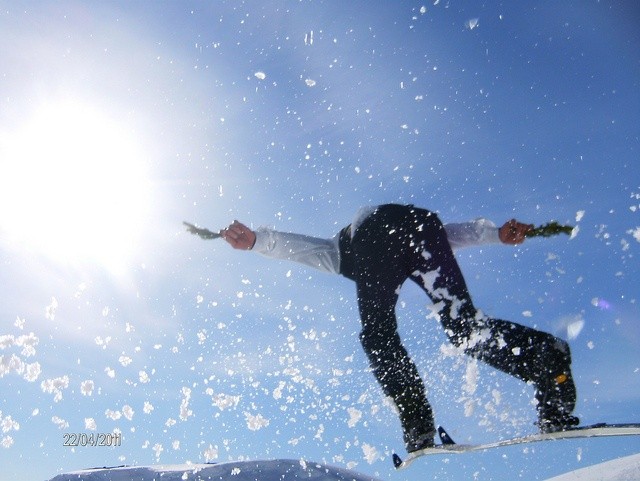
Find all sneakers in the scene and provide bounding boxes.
[404,421,439,453]
[535,338,577,417]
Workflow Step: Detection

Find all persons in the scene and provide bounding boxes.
[217,204,579,452]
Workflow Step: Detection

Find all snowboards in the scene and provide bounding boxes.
[393,416,640,473]
